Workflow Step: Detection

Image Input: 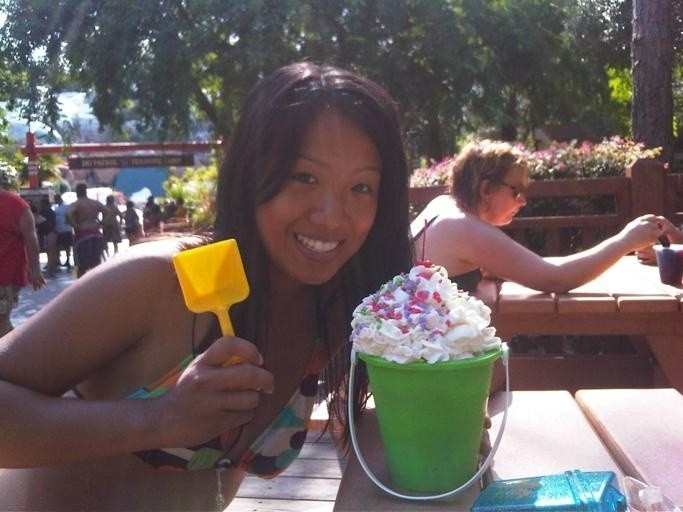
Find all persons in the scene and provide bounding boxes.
[1,60,494,510]
[635,214,682,265]
[411,137,663,296]
[28,182,194,279]
[0,188,47,339]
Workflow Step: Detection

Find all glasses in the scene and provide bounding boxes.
[499,180,522,199]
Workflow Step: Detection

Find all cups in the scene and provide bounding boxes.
[651,242,682,285]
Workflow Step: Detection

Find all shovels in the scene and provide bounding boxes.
[173,238,250,370]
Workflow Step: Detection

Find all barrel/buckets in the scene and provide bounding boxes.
[346,342,510,503]
